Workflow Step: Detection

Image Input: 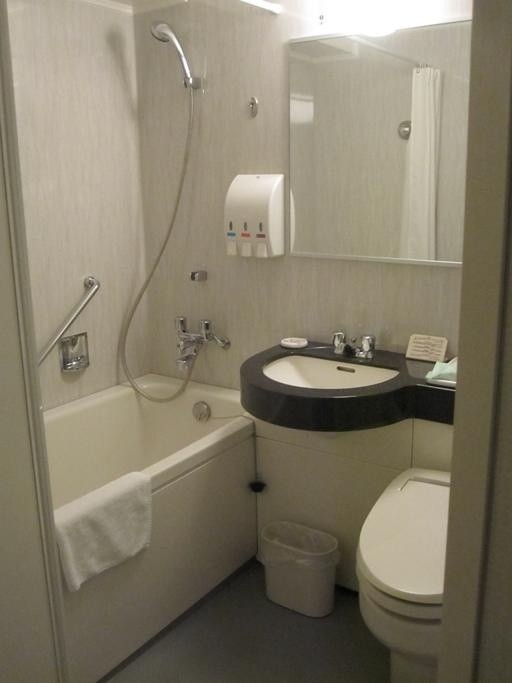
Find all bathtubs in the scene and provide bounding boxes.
[43,373,257,683]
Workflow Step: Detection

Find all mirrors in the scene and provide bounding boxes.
[288,19,472,269]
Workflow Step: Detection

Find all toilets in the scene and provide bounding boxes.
[356,468,450,683]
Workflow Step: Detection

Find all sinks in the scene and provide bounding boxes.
[261,353,399,390]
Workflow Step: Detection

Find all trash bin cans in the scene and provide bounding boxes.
[261,520,339,618]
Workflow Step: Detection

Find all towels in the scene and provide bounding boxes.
[425,356,457,388]
[52,471,153,593]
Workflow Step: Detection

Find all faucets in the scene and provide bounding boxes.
[176,353,199,370]
[333,339,358,357]
